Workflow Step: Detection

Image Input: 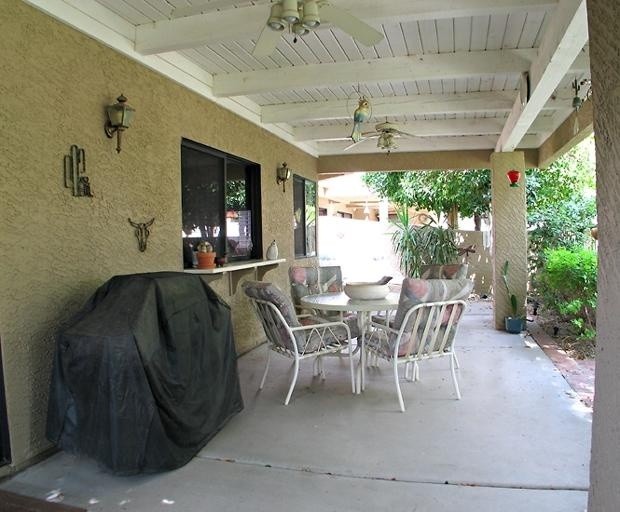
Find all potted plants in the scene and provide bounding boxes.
[500,259,526,333]
[196,241,217,269]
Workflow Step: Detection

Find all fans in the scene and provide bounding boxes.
[343,116,421,160]
[251,0,385,57]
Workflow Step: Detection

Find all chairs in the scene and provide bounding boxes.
[239,263,475,413]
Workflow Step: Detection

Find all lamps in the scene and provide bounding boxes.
[104,93,136,154]
[377,135,399,150]
[266,0,320,37]
[505,170,521,187]
[277,162,291,193]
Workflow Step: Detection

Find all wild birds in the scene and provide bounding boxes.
[349,99,373,144]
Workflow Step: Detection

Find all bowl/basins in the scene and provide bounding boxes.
[344,281,390,300]
